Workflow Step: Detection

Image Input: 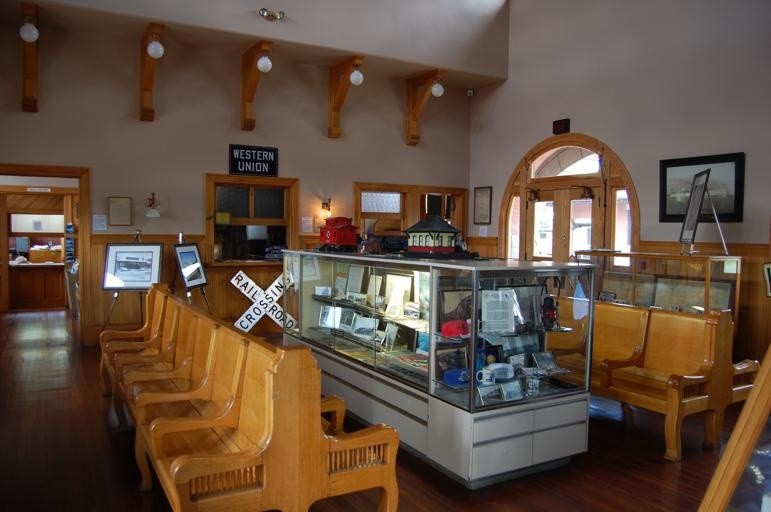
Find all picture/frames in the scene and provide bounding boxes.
[175,243,207,288]
[101,243,163,291]
[659,152,745,223]
[598,271,658,308]
[107,196,132,226]
[473,186,492,225]
[678,168,730,256]
[652,273,736,316]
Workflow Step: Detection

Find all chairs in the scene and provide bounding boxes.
[544,294,761,462]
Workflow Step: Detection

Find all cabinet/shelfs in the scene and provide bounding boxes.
[9,262,69,309]
[575,248,742,338]
[281,249,601,491]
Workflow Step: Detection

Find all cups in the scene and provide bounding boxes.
[475,367,495,386]
[466,318,480,335]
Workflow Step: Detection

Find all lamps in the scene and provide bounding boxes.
[19,14,38,43]
[321,202,330,217]
[431,78,444,98]
[349,64,364,86]
[257,50,272,73]
[148,32,165,58]
